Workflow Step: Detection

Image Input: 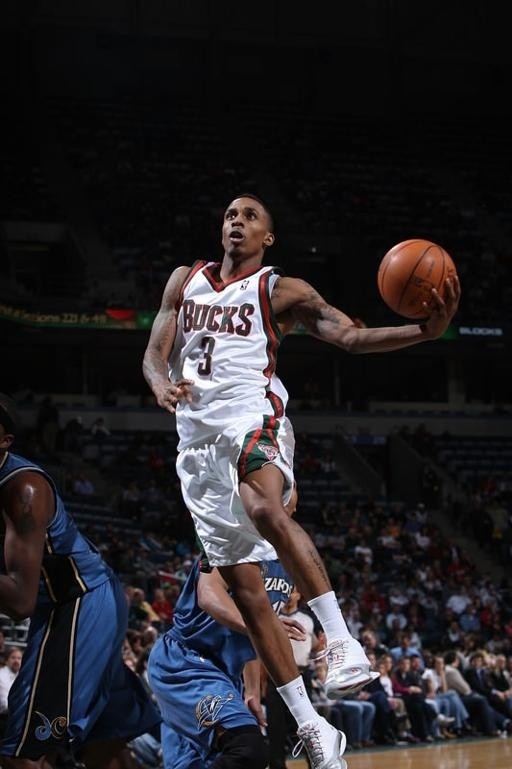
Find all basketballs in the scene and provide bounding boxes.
[377,239,458,320]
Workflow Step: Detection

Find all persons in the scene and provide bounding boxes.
[141,196,461,766]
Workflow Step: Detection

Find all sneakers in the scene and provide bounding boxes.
[297,716,347,769]
[324,636,381,700]
